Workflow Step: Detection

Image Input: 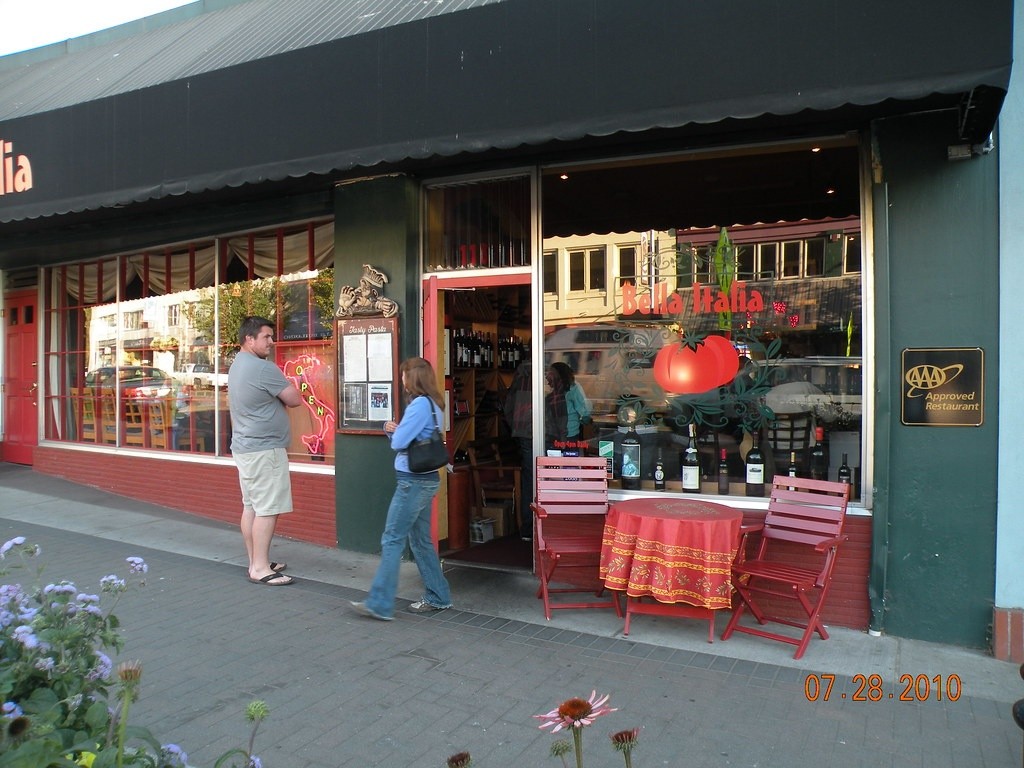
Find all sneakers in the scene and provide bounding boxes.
[348,601,394,620]
[409,600,439,612]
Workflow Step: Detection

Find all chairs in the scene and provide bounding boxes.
[71,385,204,453]
[465,439,526,534]
[526,457,623,619]
[720,474,851,660]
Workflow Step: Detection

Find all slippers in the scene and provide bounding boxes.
[249,572,294,585]
[247,562,287,576]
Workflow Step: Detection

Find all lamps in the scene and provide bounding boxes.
[104,342,111,357]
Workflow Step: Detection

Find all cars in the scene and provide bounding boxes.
[85,367,190,421]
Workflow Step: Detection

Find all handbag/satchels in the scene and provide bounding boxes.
[408,395,450,473]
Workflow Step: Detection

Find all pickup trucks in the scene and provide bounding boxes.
[171,364,229,388]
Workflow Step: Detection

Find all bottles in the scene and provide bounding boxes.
[809,427,828,495]
[621,410,642,490]
[654,457,665,492]
[838,453,850,500]
[453,328,525,369]
[745,431,765,497]
[786,451,798,491]
[682,424,701,493]
[718,448,729,494]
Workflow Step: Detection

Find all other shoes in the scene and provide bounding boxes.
[522,535,533,542]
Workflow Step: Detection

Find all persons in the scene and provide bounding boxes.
[348,357,453,622]
[505,337,594,542]
[228,316,300,587]
[157,370,214,454]
[667,357,842,478]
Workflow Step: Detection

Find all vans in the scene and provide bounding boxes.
[544,325,678,426]
[662,356,863,436]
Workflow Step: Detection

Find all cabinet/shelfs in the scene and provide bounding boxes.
[445,287,532,484]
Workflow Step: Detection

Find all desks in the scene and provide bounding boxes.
[599,498,743,643]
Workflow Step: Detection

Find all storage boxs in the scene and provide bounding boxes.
[469,501,514,543]
